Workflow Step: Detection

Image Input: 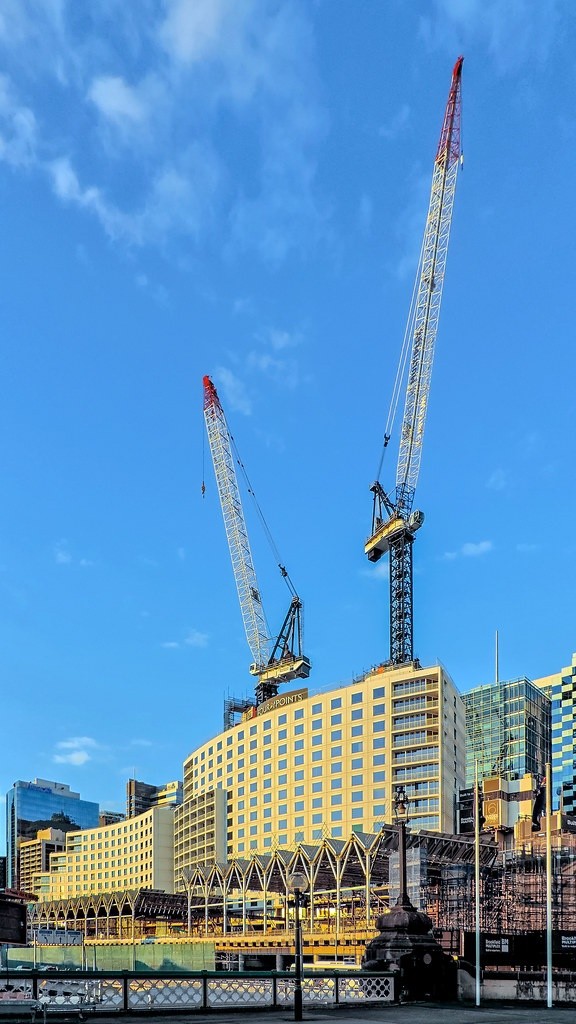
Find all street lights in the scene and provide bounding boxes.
[393,785,413,906]
[284,872,308,1021]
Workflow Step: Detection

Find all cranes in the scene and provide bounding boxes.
[362,52,465,665]
[201,374,312,709]
